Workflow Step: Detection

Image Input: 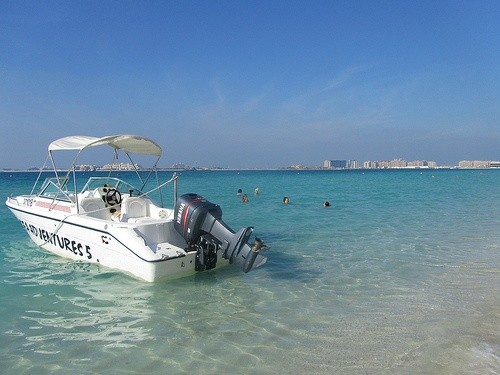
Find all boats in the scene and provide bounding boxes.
[5,134,269,283]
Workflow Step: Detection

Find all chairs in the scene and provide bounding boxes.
[111,196,147,222]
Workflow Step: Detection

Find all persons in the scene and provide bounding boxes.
[237,189,243,195]
[283,197,289,204]
[324,202,329,206]
[240,194,248,202]
[255,187,259,194]
